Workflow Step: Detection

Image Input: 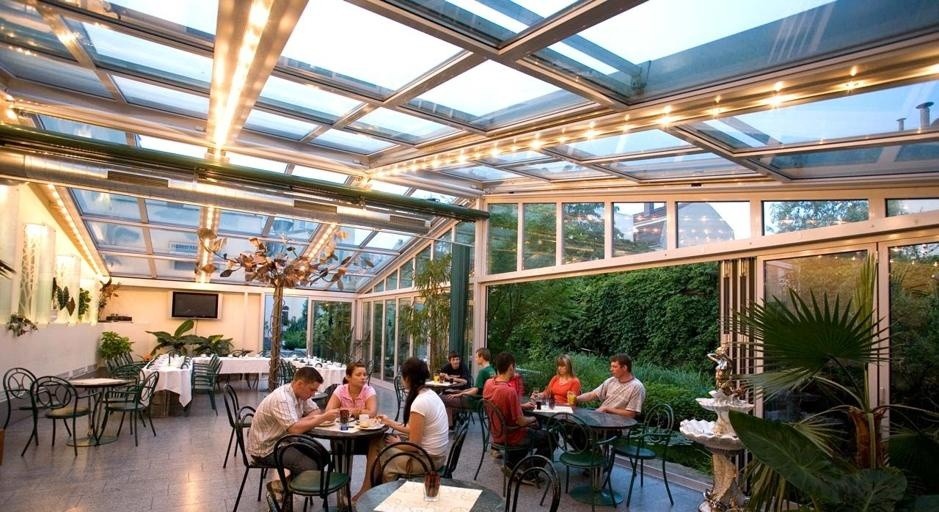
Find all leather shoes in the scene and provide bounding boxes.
[342,495,357,510]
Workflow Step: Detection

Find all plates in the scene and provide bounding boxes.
[318,420,336,426]
[359,423,385,431]
[336,417,353,422]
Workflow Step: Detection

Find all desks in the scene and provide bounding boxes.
[419,380,468,420]
[356,476,506,512]
[516,398,639,512]
[307,414,387,512]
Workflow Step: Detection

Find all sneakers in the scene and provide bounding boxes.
[266,480,283,511]
[492,450,545,485]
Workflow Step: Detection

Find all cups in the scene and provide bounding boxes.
[359,414,369,427]
[339,409,349,430]
[424,470,441,501]
[434,372,453,384]
[533,387,539,397]
[536,402,542,409]
[566,390,575,404]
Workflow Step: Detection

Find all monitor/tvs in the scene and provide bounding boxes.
[168,290,223,320]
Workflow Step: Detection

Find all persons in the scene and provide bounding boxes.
[481,352,559,487]
[568,353,647,478]
[324,360,379,508]
[439,347,499,434]
[245,367,340,511]
[490,372,526,460]
[529,353,583,411]
[348,355,450,506]
[439,349,471,391]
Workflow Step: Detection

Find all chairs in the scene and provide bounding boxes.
[399,421,472,478]
[540,412,622,512]
[1,350,225,460]
[392,375,421,434]
[472,396,540,496]
[231,404,314,510]
[369,442,435,487]
[506,454,562,512]
[602,405,676,507]
[222,385,262,468]
[274,433,353,510]
